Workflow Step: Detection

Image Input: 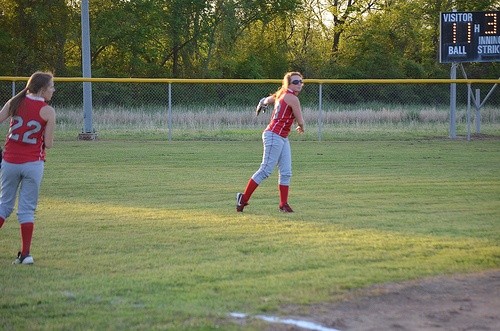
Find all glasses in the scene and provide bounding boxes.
[289,80,303,84]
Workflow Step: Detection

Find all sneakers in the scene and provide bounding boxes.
[279,204,294,212]
[12,251,33,265]
[237,193,246,212]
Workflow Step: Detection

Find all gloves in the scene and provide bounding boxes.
[256,97,268,116]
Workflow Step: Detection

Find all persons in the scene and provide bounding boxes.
[236,71,305,212]
[0,68,56,267]
